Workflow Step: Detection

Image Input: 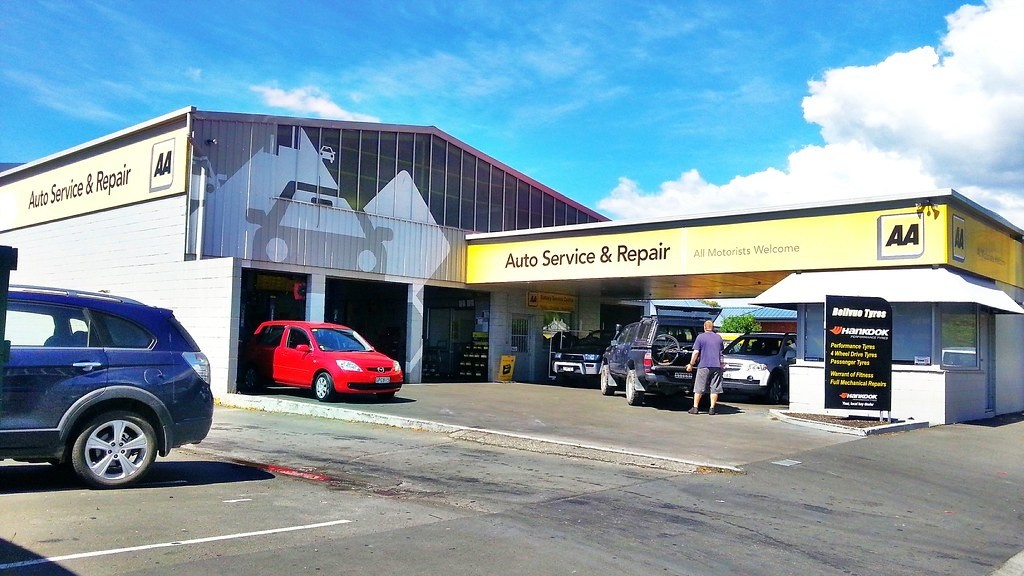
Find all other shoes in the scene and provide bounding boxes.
[708,408,716,415]
[687,407,698,414]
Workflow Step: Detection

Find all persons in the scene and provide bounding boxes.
[685,320,724,415]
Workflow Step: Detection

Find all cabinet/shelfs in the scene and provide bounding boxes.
[456,345,489,377]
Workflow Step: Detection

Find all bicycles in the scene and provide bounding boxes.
[652,334,694,366]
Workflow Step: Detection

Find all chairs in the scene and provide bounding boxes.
[42,331,88,347]
[748,341,763,355]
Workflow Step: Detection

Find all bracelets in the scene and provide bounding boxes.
[690,361,696,366]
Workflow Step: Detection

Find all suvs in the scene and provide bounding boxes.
[0,284,214,490]
[720,330,797,405]
[600,315,711,406]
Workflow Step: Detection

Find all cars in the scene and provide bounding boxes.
[238,320,403,401]
[550,330,618,383]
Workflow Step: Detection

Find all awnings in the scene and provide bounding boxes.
[748,267,1024,315]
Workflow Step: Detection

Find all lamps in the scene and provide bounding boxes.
[206,138,218,145]
